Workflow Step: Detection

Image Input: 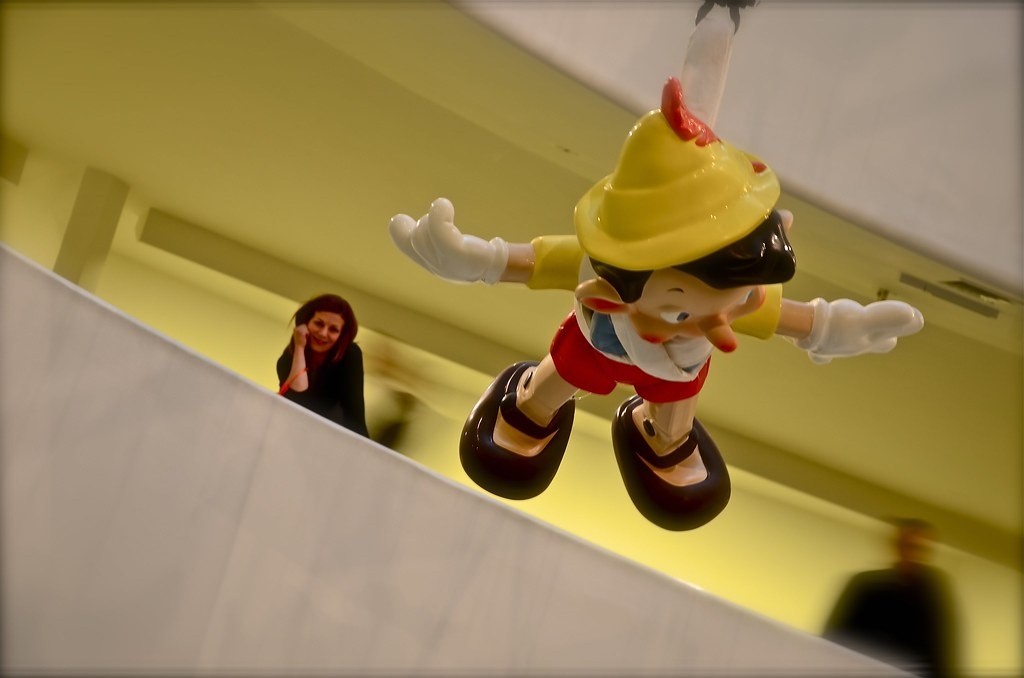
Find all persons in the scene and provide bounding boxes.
[820,517,954,678]
[387,76,926,532]
[277,294,368,437]
